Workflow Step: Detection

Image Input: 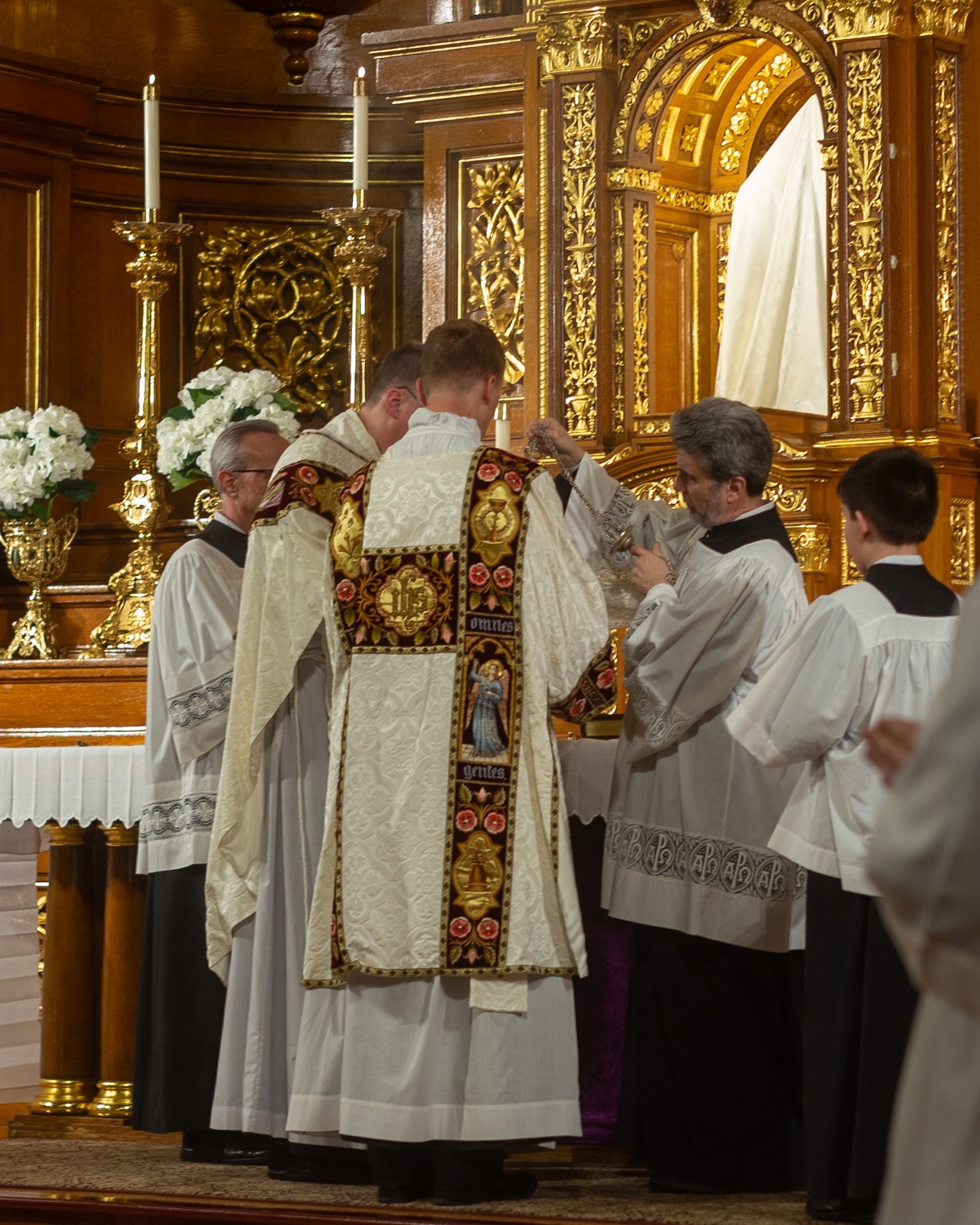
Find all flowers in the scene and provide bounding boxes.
[0,402,100,527]
[156,367,301,492]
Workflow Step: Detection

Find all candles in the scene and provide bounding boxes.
[142,74,160,209]
[494,402,510,451]
[352,66,369,190]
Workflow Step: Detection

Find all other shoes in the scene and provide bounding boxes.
[807,1197,877,1223]
[377,1173,434,1203]
[647,1165,791,1194]
[266,1153,370,1184]
[433,1168,539,1207]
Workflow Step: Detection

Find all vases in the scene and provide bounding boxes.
[0,507,79,660]
[194,486,222,531]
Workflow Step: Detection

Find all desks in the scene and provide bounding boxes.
[0,658,148,1116]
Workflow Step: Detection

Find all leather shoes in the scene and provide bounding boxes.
[178,1129,272,1165]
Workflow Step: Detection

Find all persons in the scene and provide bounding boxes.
[860,571,980,1225]
[728,449,966,1224]
[140,421,290,1164]
[522,393,813,1198]
[326,319,615,1208]
[251,342,423,1198]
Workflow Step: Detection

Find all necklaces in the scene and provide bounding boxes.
[525,424,676,585]
